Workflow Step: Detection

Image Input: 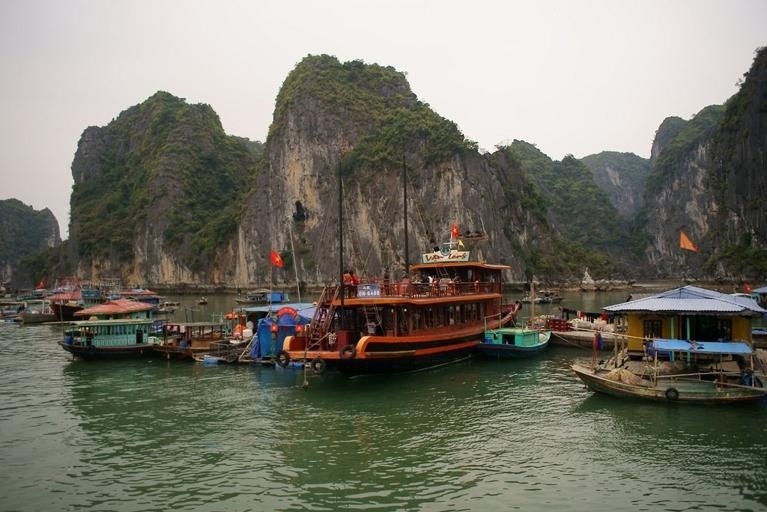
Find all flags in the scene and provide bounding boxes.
[679,233,696,251]
[451,222,459,238]
[270,249,282,267]
[36,280,45,289]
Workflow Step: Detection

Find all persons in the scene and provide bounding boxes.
[343,269,353,298]
[349,270,359,297]
[474,278,480,293]
[411,272,417,283]
[426,273,433,292]
[431,279,439,295]
